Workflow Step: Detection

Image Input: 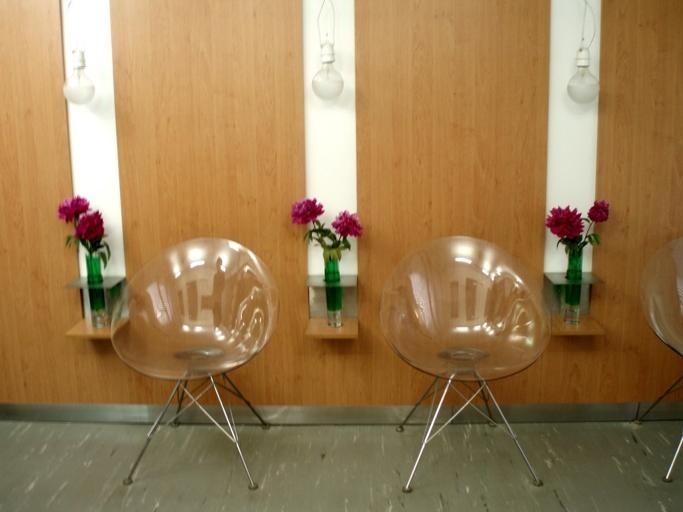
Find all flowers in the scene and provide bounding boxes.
[290,199,363,260]
[545,200,610,255]
[57,198,110,269]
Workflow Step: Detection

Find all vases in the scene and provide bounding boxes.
[85,256,107,325]
[562,249,584,325]
[323,258,342,327]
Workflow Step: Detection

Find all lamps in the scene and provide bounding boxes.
[312,1,343,100]
[568,0,600,104]
[63,2,94,105]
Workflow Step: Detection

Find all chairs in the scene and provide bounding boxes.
[637,239,683,485]
[109,238,278,491]
[380,235,551,494]
